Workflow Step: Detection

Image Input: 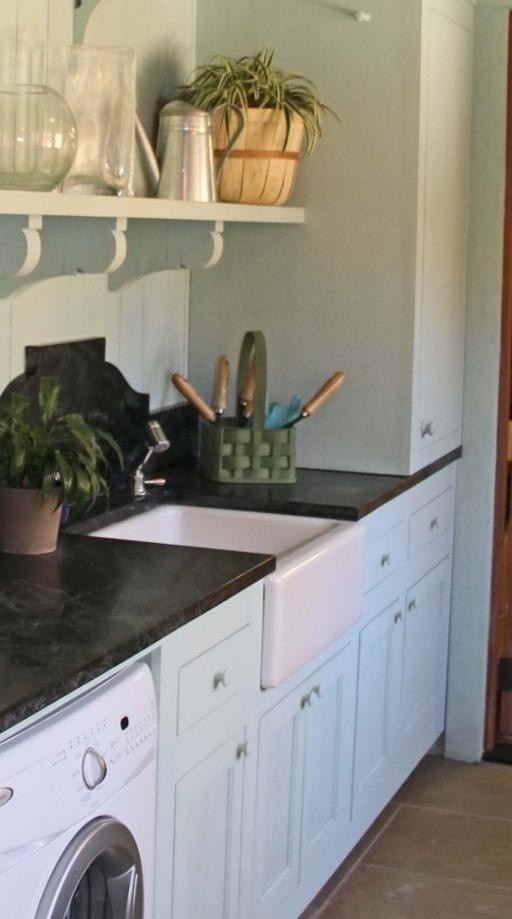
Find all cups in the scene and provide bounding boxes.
[62,40,135,196]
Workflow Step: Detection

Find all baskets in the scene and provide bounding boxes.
[191,329,300,488]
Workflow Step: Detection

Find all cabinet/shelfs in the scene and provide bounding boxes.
[189,0,476,478]
[147,458,458,919]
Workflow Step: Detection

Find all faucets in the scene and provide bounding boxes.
[126,419,169,500]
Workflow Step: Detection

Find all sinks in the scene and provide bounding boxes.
[87,500,368,690]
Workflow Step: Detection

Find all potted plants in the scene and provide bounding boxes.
[0,372,126,557]
[170,44,344,207]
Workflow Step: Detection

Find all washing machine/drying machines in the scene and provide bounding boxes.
[1,663,152,918]
